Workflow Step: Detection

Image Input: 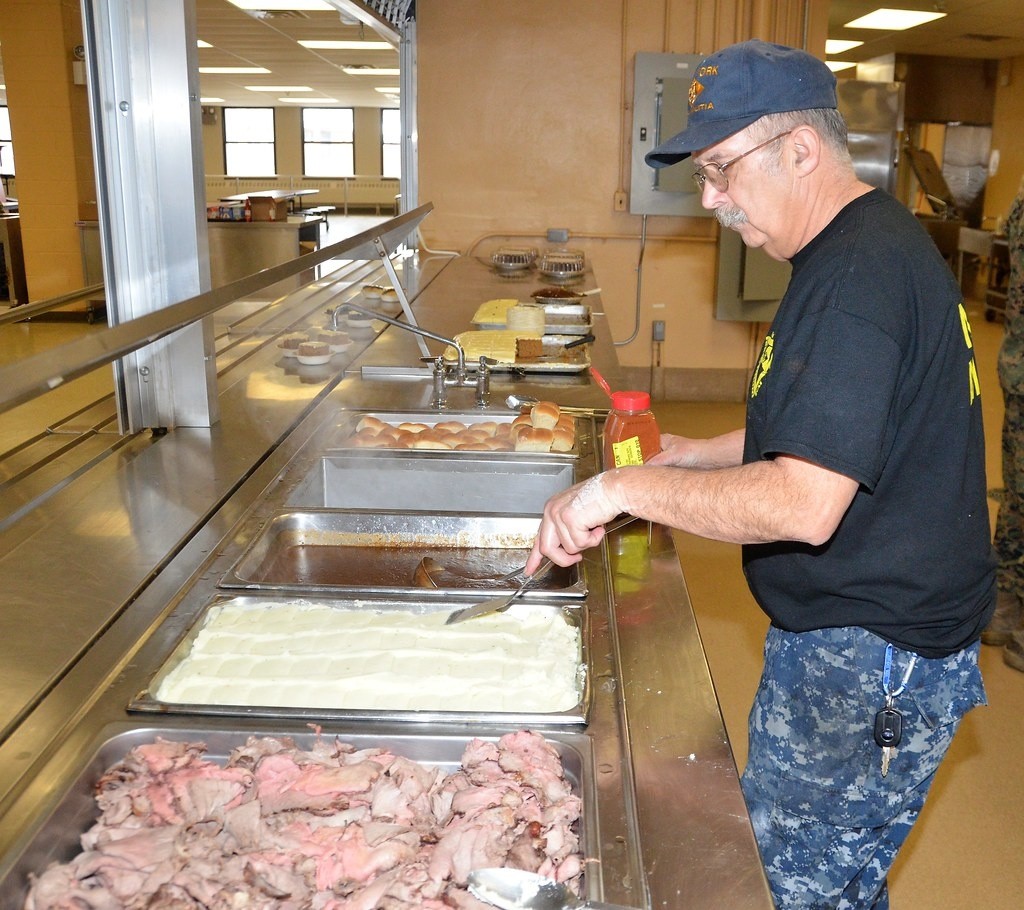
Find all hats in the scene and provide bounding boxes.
[645,38,837,169]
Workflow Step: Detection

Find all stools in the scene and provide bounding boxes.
[293,206,335,231]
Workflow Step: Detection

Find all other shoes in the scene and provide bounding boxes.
[981,629,1007,646]
[1002,644,1024,672]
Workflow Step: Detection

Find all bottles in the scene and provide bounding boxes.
[245,199,252,221]
[590,367,661,473]
[996,213,1003,236]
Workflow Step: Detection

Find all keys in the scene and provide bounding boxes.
[874,710,902,777]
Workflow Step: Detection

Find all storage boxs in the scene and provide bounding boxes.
[248,196,286,222]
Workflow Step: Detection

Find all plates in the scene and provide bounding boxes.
[469,302,593,335]
[533,296,587,305]
[492,261,531,271]
[538,267,583,277]
[444,334,591,373]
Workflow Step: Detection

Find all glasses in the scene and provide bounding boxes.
[692,131,792,193]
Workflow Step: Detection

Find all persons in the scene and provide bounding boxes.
[524,38,994,910]
[979,187,1024,671]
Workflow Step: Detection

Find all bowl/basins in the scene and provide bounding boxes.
[277,344,299,358]
[330,339,354,353]
[381,301,407,312]
[343,315,378,327]
[361,297,382,308]
[294,350,336,366]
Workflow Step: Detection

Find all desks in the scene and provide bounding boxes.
[216,189,319,214]
[208,215,323,301]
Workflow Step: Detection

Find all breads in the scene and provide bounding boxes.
[342,400,577,452]
[443,328,543,365]
